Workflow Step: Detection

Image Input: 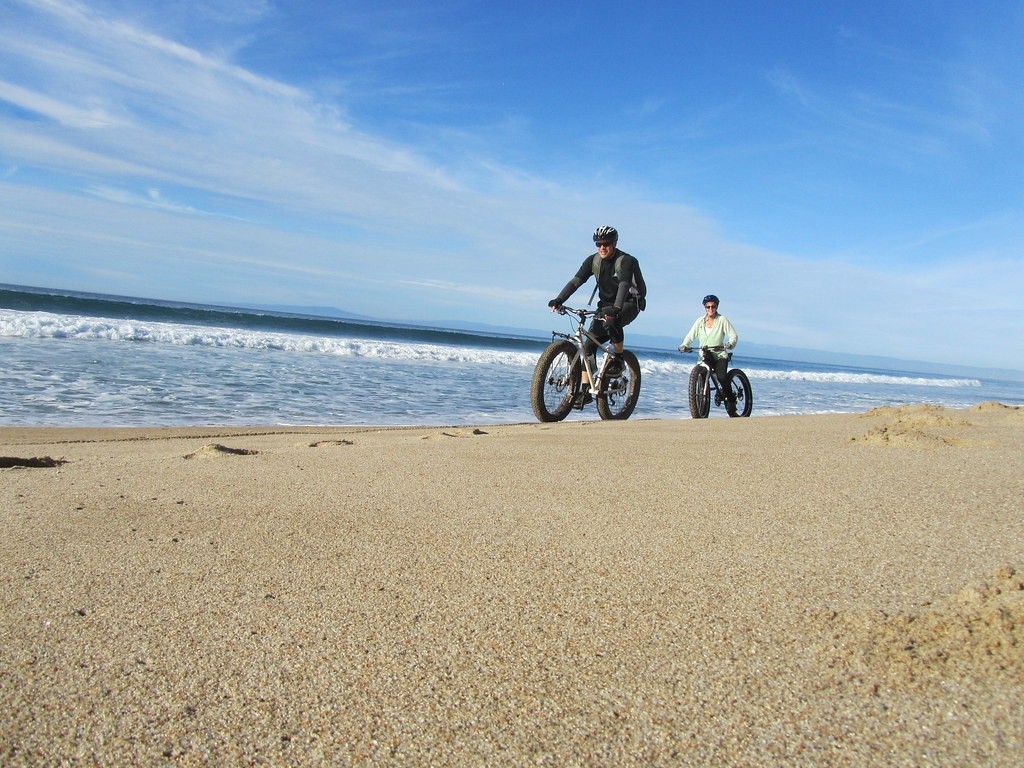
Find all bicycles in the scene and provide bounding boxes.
[677,345,754,420]
[531,303,643,422]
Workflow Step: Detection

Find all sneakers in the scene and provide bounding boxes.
[573,392,593,409]
[605,357,627,379]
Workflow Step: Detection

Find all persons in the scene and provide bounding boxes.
[679,295,738,414]
[548,225,640,405]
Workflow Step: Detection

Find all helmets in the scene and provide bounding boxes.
[592,225,618,240]
[703,295,720,305]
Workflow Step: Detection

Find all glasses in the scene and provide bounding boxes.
[596,241,612,247]
[705,306,716,309]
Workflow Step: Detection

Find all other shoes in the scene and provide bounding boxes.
[726,397,736,414]
